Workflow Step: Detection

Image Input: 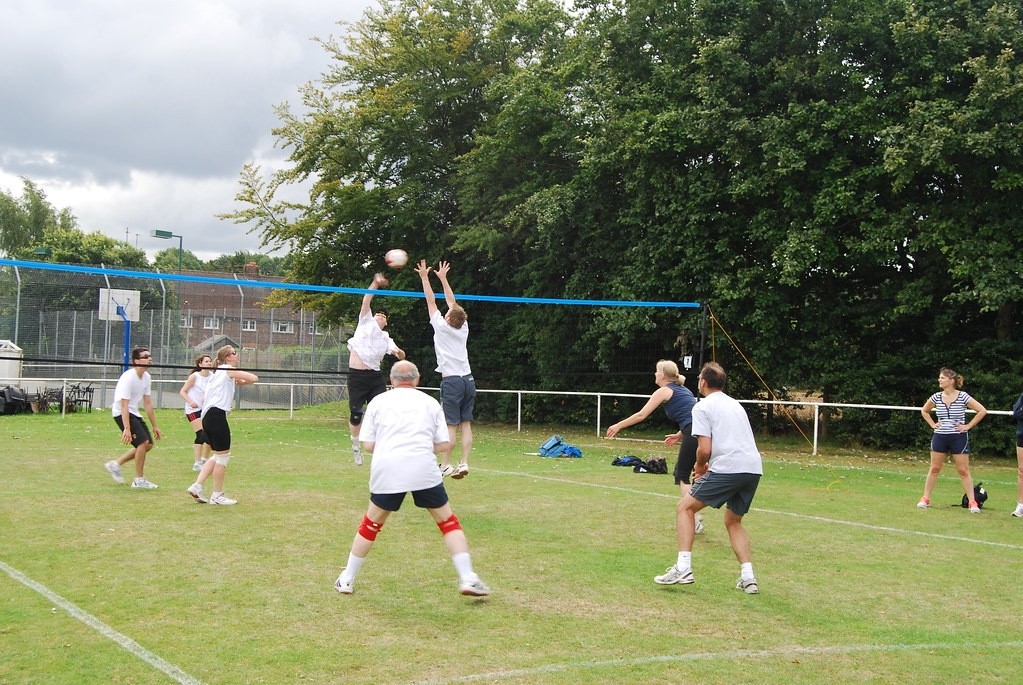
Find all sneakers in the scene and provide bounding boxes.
[653,563,695,586]
[458,574,490,596]
[187,482,208,504]
[695,515,704,535]
[917,496,929,508]
[451,464,469,480]
[209,492,237,505]
[968,500,981,513]
[438,464,455,480]
[1011,503,1023,517]
[736,578,758,595]
[131,476,159,488]
[193,457,206,473]
[352,443,364,468]
[334,571,355,594]
[104,460,125,485]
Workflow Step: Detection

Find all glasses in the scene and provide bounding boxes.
[135,354,152,359]
[375,313,387,318]
[226,350,237,356]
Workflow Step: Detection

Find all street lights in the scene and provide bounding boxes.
[148,228,183,390]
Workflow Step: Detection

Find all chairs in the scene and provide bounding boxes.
[25,385,94,414]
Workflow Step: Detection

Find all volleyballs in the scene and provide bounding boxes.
[385,248,408,269]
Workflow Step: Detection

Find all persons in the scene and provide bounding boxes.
[347,273,406,466]
[1011,392,1023,517]
[335,359,490,596]
[180,355,214,472]
[414,260,476,479]
[104,347,161,489]
[606,360,704,534]
[655,363,762,594]
[916,369,987,513]
[187,345,259,505]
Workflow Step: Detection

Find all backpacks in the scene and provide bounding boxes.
[962,482,987,508]
